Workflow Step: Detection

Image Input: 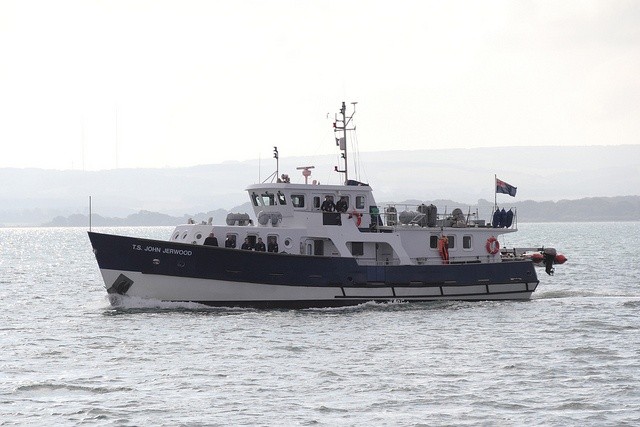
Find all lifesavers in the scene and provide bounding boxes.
[486,236,500,254]
[349,211,361,225]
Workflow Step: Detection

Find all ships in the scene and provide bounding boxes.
[86,101,567,311]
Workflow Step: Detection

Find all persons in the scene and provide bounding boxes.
[226,235,235,248]
[240,237,253,251]
[268,237,278,252]
[202,232,219,246]
[335,196,348,212]
[320,195,335,212]
[255,237,266,251]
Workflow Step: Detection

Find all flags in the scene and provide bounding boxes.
[497,178,517,197]
